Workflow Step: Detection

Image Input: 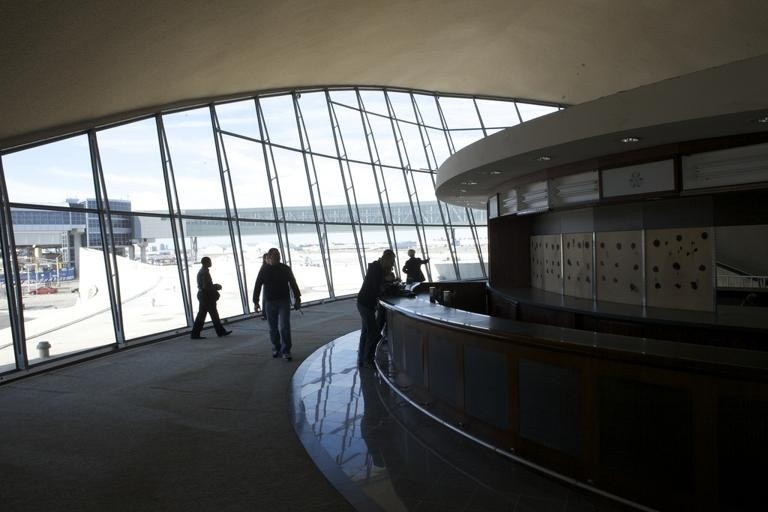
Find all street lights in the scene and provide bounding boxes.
[0,244,68,286]
[75,197,88,247]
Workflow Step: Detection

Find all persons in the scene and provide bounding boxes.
[357,250,402,369]
[402,249,430,283]
[190,256,232,339]
[253,248,301,361]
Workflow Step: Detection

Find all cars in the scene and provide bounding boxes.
[27,285,58,295]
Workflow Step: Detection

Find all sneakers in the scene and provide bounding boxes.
[357,358,376,368]
[272,349,279,357]
[282,353,292,359]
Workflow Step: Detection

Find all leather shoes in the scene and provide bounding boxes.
[191,335,206,339]
[218,331,232,337]
